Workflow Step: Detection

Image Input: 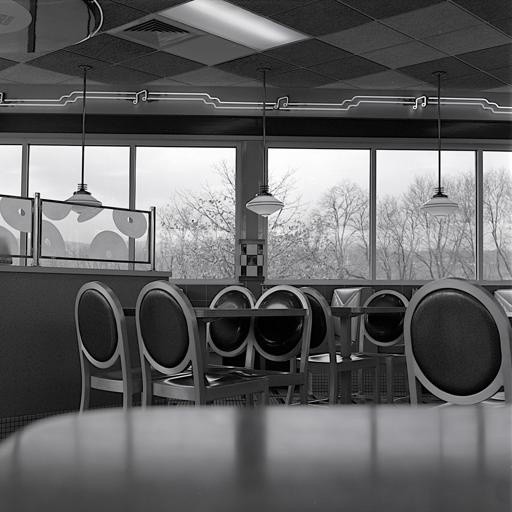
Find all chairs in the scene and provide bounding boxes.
[74,279,167,412]
[245,282,313,412]
[203,284,256,375]
[137,279,270,411]
[299,280,512,409]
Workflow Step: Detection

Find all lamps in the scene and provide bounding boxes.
[421,72,460,220]
[245,73,284,217]
[61,63,103,223]
